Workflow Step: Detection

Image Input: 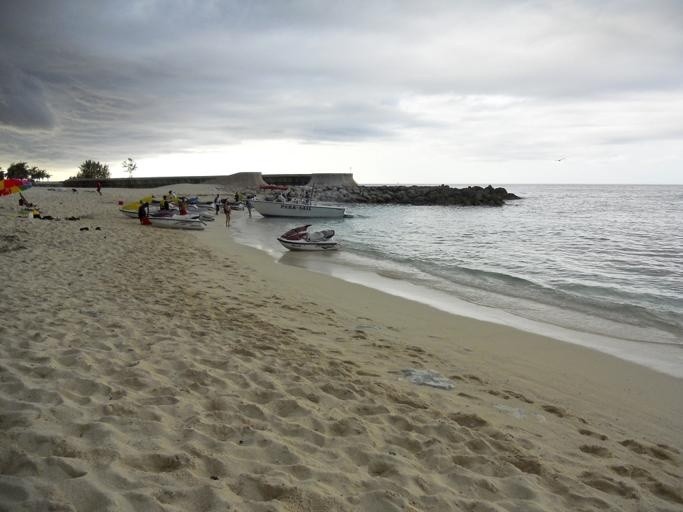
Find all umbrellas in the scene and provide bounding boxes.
[0,176,33,203]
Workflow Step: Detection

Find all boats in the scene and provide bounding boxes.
[276,234,337,251]
[118,197,243,230]
[246,199,346,219]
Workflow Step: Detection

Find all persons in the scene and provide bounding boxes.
[167,190,177,204]
[159,195,176,211]
[138,202,149,225]
[95,180,102,196]
[18,196,36,208]
[272,188,310,205]
[179,197,192,215]
[212,191,253,228]
